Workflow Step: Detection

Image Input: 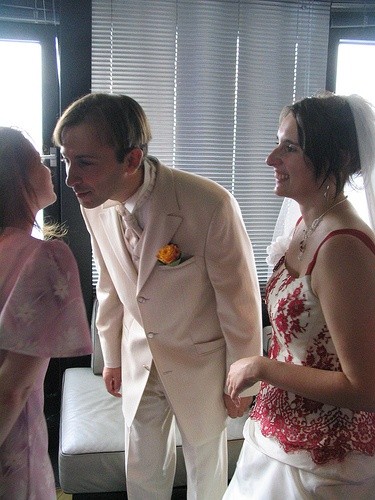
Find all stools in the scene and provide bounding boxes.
[55,361,252,499]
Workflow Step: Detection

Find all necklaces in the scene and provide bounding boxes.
[295,194,346,263]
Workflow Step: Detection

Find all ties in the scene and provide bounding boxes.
[103,156,158,247]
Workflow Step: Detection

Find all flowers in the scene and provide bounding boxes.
[156,241,184,267]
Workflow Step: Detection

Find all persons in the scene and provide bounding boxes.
[225,93,375,500]
[51,90,265,500]
[0,126,94,500]
